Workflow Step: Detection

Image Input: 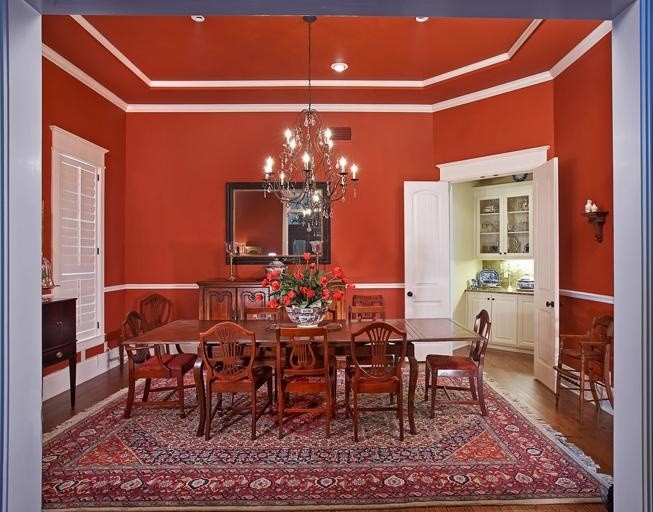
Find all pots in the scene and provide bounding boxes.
[517,274,534,288]
[482,205,498,213]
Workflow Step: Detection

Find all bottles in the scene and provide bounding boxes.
[522,199,527,210]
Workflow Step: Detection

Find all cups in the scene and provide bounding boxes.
[522,222,528,231]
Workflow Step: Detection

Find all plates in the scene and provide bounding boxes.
[478,269,499,286]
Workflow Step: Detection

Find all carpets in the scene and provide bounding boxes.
[41,365,613,509]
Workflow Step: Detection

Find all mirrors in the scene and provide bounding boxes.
[223,180,330,265]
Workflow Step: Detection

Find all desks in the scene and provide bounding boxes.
[120,316,488,436]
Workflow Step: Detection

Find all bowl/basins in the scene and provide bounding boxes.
[482,246,497,253]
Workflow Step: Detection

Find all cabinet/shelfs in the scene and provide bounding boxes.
[471,180,533,261]
[516,294,535,354]
[464,290,517,357]
[193,279,346,322]
[42,294,78,408]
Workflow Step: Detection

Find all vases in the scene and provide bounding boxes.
[284,299,333,328]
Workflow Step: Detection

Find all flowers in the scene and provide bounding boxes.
[252,252,357,310]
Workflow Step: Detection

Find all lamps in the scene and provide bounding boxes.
[260,16,359,233]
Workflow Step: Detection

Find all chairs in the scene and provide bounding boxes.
[138,295,174,329]
[119,310,200,421]
[352,294,385,307]
[342,320,409,440]
[552,314,612,424]
[347,304,387,322]
[197,321,275,441]
[423,307,491,421]
[273,323,340,440]
